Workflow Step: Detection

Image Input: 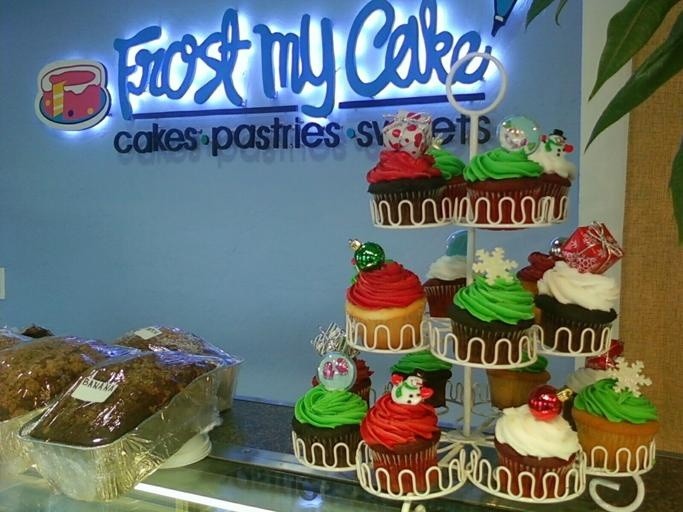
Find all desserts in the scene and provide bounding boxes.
[292,110,660,497]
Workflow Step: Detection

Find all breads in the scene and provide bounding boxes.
[0,323,235,445]
